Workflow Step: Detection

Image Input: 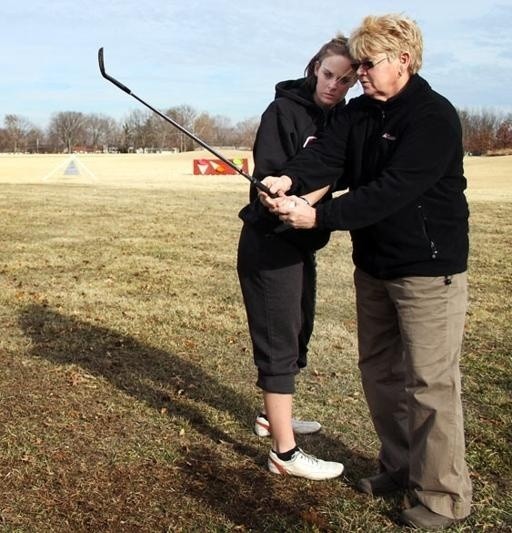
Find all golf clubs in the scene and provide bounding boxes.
[98,47,280,201]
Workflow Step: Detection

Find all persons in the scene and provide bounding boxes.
[255,12,472,530]
[237,31,344,481]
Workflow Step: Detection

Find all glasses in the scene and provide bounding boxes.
[350,57,388,71]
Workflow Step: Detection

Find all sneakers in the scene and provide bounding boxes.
[361,469,406,494]
[269,447,344,481]
[255,413,320,438]
[401,503,466,532]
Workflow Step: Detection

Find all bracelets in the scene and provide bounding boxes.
[298,198,311,208]
